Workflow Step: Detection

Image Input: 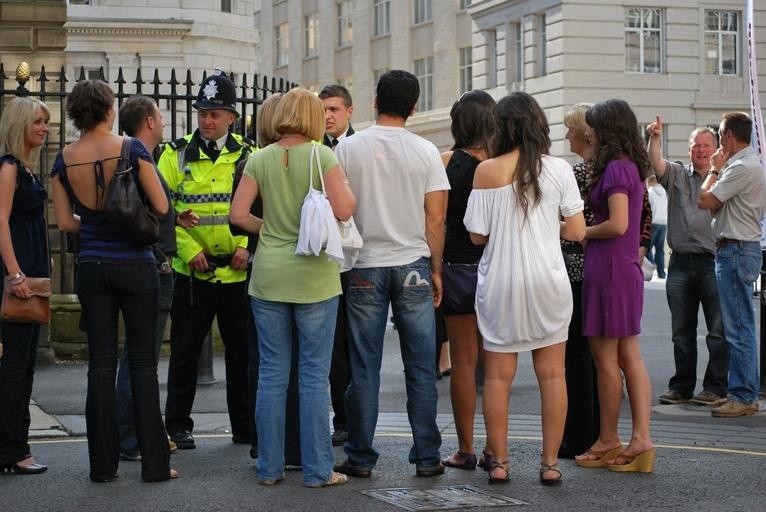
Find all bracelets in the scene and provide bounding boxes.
[706,170,719,174]
[7,272,26,285]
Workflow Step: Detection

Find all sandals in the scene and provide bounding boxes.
[540,462,562,486]
[477,451,510,484]
[441,450,477,470]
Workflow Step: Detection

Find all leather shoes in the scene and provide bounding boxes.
[171,427,196,449]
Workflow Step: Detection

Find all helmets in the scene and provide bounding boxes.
[191,75,240,119]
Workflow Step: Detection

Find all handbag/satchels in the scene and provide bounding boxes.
[0,276,53,324]
[300,187,363,254]
[101,155,160,245]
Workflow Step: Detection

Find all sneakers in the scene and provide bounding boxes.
[658,390,693,404]
[416,460,446,476]
[232,428,374,487]
[540,443,578,459]
[117,445,142,460]
[693,389,728,404]
[716,396,760,412]
[711,398,755,416]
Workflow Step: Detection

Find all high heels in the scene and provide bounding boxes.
[31,464,48,471]
[0,464,44,478]
[576,445,623,467]
[608,449,654,473]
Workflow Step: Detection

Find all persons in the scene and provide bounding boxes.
[116,94,177,461]
[1,95,52,473]
[463,91,585,485]
[590,99,667,472]
[648,115,727,406]
[158,75,257,448]
[698,112,765,417]
[541,103,614,460]
[51,78,178,481]
[336,71,444,478]
[235,83,360,487]
[439,89,501,469]
